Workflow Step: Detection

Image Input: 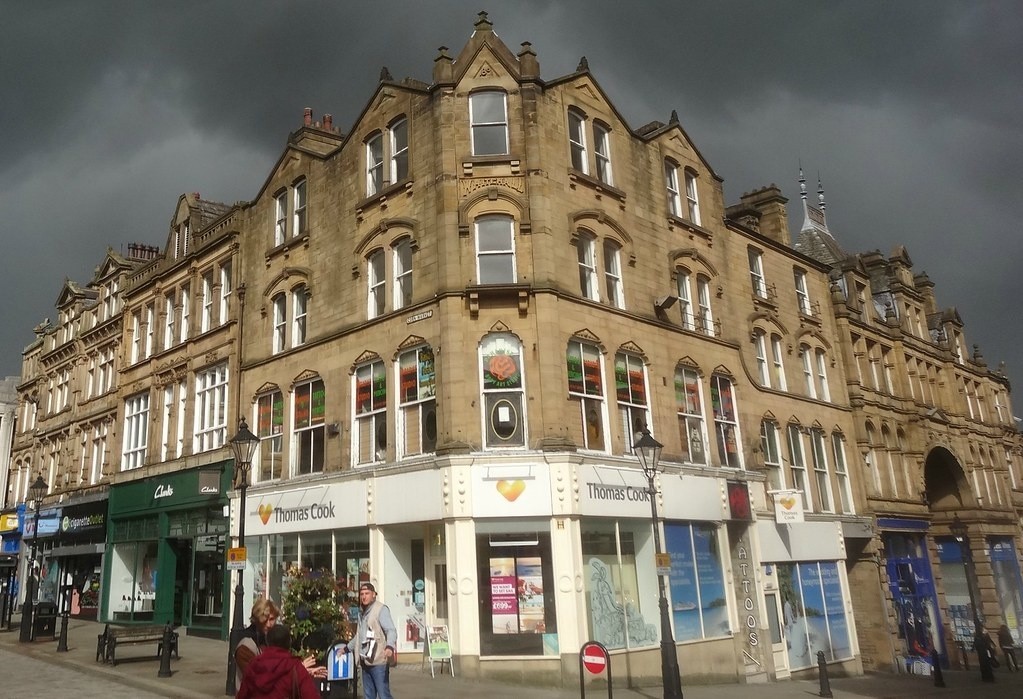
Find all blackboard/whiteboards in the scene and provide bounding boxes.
[426,625,452,659]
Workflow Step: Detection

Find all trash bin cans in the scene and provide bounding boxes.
[33,601,58,640]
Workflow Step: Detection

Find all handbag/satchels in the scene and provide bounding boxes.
[359,626,375,659]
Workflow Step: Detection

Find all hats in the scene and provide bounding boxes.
[360,583,377,595]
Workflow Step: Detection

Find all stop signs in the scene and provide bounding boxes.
[584,644,607,674]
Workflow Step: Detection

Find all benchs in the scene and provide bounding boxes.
[96,623,179,667]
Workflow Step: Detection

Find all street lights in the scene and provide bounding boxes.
[225,414,262,695]
[18,471,50,643]
[948,510,995,682]
[631,422,685,699]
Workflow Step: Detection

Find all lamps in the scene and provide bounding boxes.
[655,294,678,308]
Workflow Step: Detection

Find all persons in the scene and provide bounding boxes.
[337,582,397,699]
[970,619,1019,682]
[8,574,19,615]
[234,599,328,699]
